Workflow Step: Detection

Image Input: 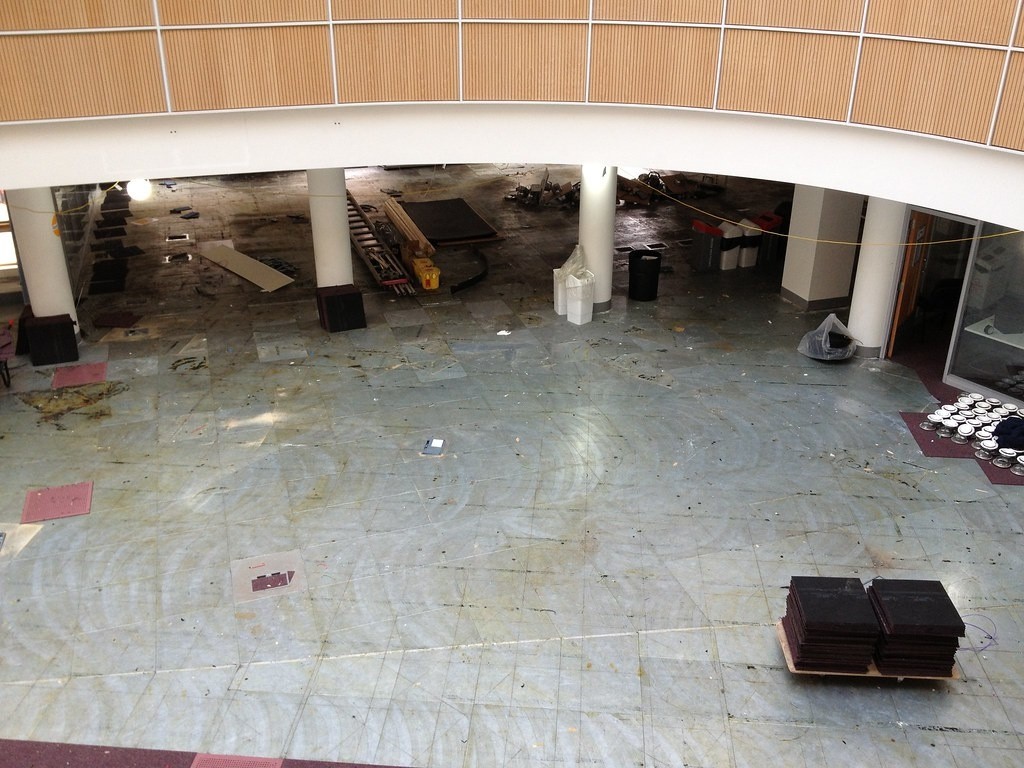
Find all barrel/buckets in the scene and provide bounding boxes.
[628,248,661,302]
[412,257,441,290]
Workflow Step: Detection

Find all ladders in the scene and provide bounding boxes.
[346,189,415,291]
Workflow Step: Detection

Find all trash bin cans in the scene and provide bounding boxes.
[736,218,762,268]
[718,221,743,271]
[628,249,662,302]
[693,220,722,272]
[753,212,780,264]
